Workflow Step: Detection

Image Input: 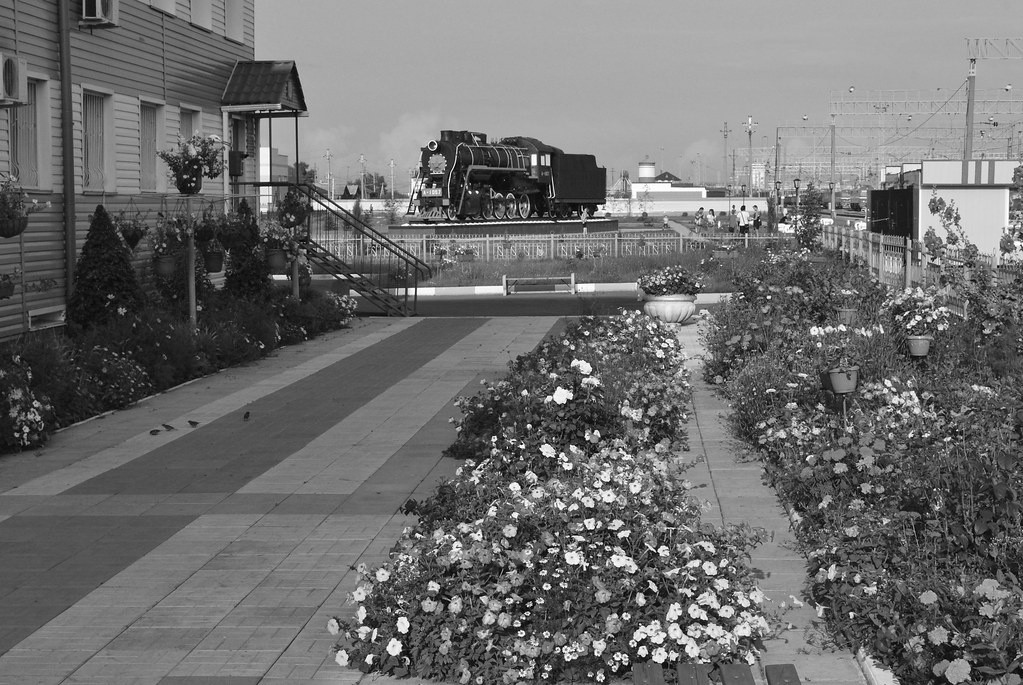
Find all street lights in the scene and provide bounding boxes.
[358,154,367,199]
[775,180,782,230]
[727,185,732,218]
[742,114,758,198]
[388,159,397,199]
[828,182,835,218]
[719,121,732,194]
[741,184,746,206]
[322,148,333,198]
[793,179,801,237]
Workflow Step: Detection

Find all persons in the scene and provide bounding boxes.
[580,206,589,233]
[705,209,717,236]
[662,215,669,229]
[695,207,705,234]
[736,205,751,244]
[779,213,793,224]
[729,210,737,236]
[750,204,760,242]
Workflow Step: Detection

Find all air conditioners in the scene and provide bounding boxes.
[0,52,29,104]
[82,0,119,26]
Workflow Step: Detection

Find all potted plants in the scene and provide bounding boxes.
[197,217,224,273]
[112,208,150,254]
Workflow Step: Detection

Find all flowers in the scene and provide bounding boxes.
[287,227,314,274]
[152,213,182,255]
[637,263,706,297]
[156,128,229,179]
[828,338,852,367]
[896,307,950,336]
[259,221,287,250]
[0,175,51,218]
[838,309,857,325]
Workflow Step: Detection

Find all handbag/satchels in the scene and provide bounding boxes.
[729,227,734,232]
[757,218,762,226]
[745,224,749,229]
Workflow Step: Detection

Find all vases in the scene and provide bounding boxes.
[906,336,933,355]
[643,294,696,326]
[290,270,311,289]
[177,166,202,194]
[155,256,174,276]
[267,251,286,269]
[830,366,858,393]
[0,217,28,238]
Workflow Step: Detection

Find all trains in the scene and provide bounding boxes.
[405,129,608,221]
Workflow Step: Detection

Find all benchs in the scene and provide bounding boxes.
[502,273,576,299]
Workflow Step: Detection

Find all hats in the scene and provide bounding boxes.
[731,210,736,214]
